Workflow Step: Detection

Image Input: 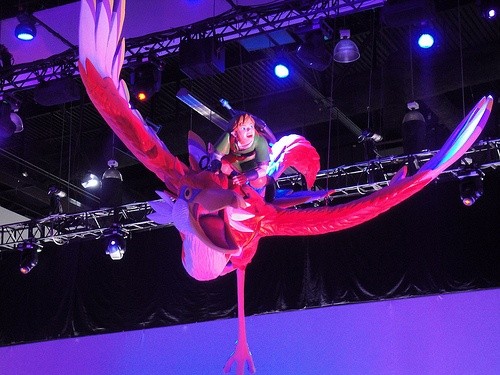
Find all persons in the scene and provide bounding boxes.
[208,114,271,186]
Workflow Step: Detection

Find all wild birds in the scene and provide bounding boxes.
[78,0,494,375]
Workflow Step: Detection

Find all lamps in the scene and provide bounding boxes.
[131,62,162,102]
[102,131,124,181]
[458,173,484,206]
[11,107,25,133]
[106,237,126,260]
[404,23,425,124]
[80,169,99,187]
[332,29,360,63]
[296,41,331,70]
[17,244,39,274]
[15,13,37,41]
[46,187,66,214]
